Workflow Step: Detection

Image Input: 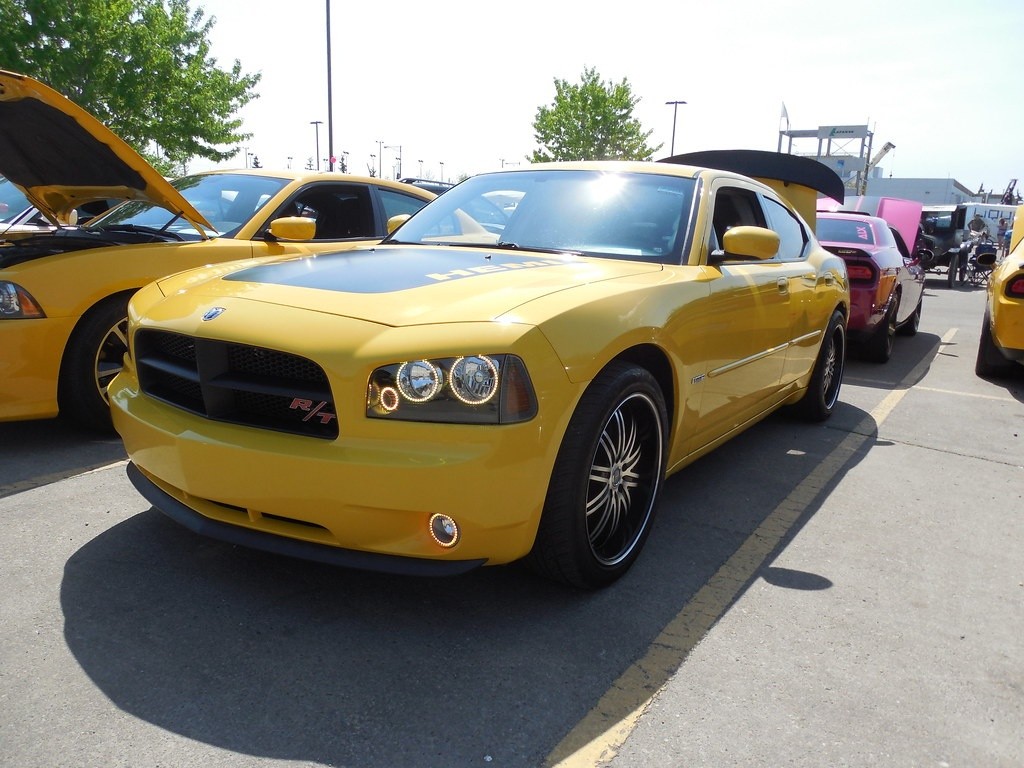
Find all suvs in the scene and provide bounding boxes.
[917,203,975,288]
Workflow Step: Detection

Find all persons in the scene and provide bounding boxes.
[967,214,1014,260]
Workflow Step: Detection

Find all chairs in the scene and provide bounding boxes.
[960,248,997,288]
[336,199,373,237]
[972,244,992,279]
[300,195,340,237]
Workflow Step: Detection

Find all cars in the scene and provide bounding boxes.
[815,196,934,363]
[0,175,258,232]
[103,147,852,592]
[0,69,487,438]
[394,177,527,234]
[975,203,1023,378]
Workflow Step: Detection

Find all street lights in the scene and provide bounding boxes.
[242,140,507,183]
[665,101,688,156]
[309,120,323,170]
